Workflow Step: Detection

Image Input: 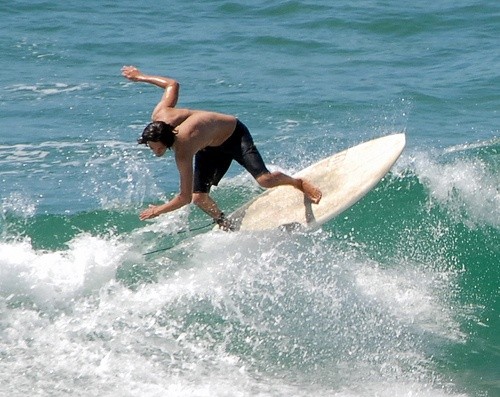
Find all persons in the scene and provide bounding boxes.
[120,64,323,232]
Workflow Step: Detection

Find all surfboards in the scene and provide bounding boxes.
[212,133,406,234]
